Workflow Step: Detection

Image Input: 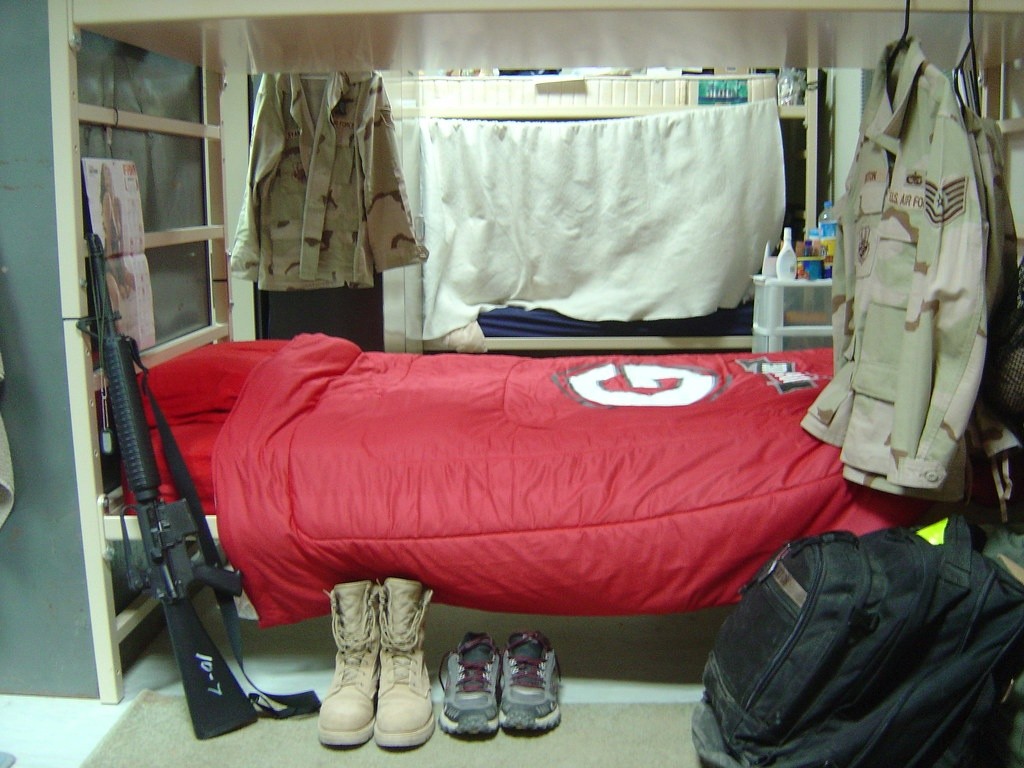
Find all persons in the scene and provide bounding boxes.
[98,163,136,333]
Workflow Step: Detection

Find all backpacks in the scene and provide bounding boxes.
[691,518,1024,768]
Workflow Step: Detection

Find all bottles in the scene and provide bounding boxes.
[801,239,817,257]
[817,201,840,238]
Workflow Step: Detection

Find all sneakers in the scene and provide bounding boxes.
[499,630,562,734]
[438,632,501,740]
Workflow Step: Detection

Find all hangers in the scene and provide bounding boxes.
[953,0,981,116]
[887,1,911,70]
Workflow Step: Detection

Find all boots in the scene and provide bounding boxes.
[317,579,380,745]
[374,577,435,747]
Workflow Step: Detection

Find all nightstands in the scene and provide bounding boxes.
[752,274,834,353]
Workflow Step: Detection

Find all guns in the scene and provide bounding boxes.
[73,233,258,739]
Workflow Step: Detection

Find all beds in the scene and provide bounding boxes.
[46,0,1024,702]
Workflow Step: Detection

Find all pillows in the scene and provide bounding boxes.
[137,340,291,429]
